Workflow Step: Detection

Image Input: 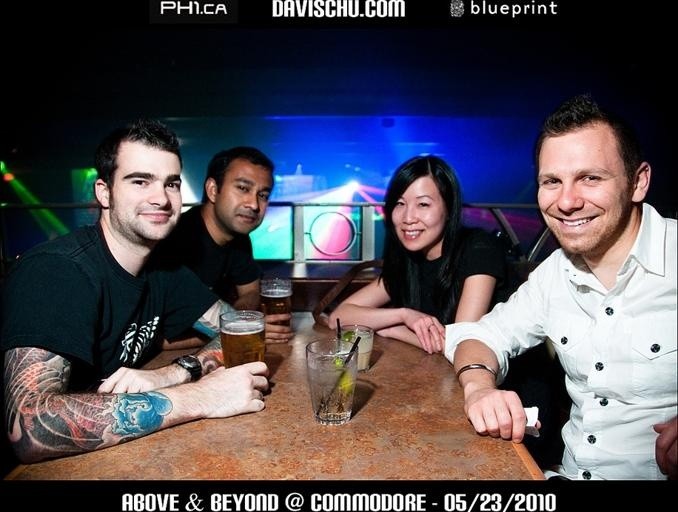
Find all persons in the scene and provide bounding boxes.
[328,155,526,355]
[0,120,268,465]
[443,93,677,481]
[140,147,294,350]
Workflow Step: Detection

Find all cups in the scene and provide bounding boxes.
[339,324,373,372]
[305,340,358,426]
[260,278,292,326]
[220,309,265,369]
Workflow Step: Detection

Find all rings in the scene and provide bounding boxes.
[427,323,435,331]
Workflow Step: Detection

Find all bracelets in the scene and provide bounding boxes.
[455,361,500,380]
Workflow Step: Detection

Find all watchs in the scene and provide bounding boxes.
[171,354,202,382]
